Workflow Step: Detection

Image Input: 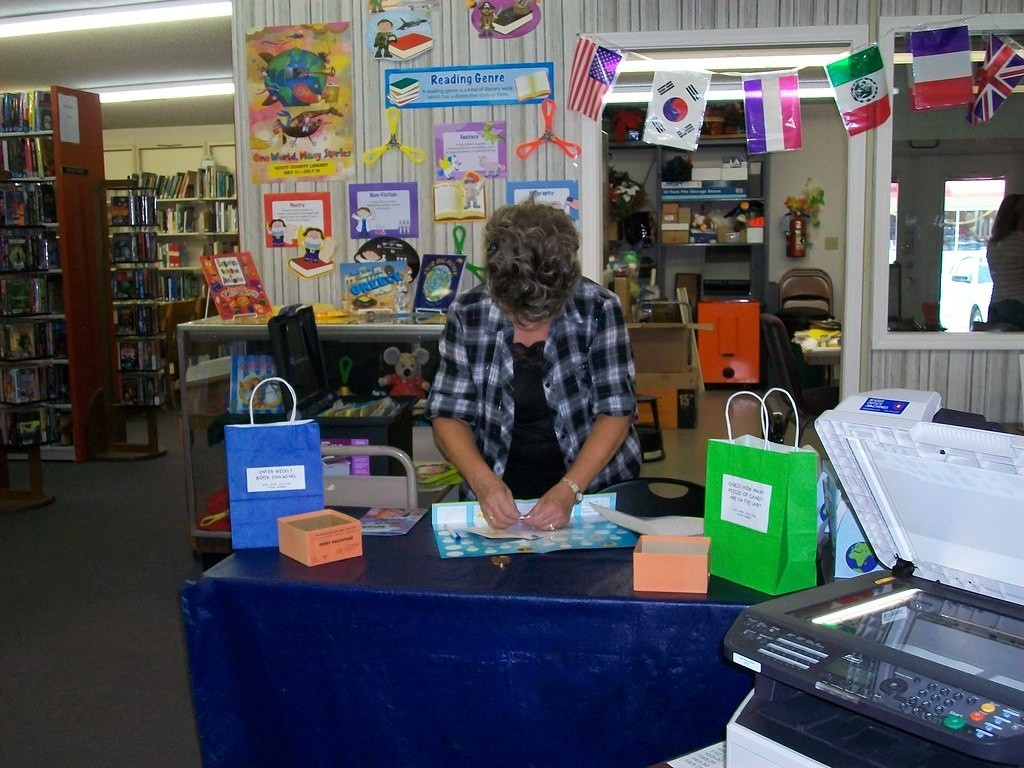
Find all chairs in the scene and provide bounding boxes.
[761,267,841,449]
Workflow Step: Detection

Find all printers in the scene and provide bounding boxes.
[652,388,1023,768]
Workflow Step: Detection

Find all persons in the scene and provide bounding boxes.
[985,193,1024,331]
[424,202,643,532]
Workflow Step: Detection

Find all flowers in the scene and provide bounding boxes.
[609,166,642,222]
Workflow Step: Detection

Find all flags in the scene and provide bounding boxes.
[566,25,1024,154]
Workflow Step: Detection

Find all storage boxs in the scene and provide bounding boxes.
[268,304,399,472]
[661,201,691,244]
[632,535,710,595]
[609,217,618,240]
[615,289,714,427]
[277,511,363,566]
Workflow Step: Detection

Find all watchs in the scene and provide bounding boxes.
[559,477,583,506]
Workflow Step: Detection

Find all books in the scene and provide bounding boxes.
[414,253,467,316]
[339,259,409,322]
[106,164,284,413]
[0,90,73,451]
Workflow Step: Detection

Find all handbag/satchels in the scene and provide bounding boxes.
[223,377,325,549]
[701,387,817,595]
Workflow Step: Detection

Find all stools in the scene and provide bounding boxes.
[0,441,56,511]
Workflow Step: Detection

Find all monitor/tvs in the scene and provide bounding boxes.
[269,303,333,423]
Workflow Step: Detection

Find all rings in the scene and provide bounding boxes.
[488,513,495,520]
[550,524,555,530]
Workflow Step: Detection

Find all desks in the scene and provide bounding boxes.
[185,509,827,768]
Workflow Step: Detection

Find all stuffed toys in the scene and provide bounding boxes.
[376,347,430,409]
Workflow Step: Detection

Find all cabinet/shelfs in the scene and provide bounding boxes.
[654,140,769,304]
[0,86,235,459]
[175,318,449,531]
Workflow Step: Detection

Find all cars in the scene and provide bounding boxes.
[944,252,994,332]
